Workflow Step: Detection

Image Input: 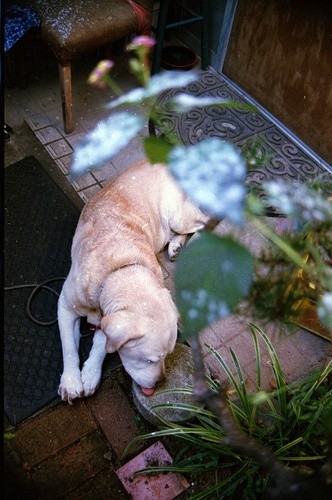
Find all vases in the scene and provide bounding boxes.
[282,224,332,341]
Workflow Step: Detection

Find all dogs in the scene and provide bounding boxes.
[57,159,224,405]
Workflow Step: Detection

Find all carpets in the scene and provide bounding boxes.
[3,154,122,430]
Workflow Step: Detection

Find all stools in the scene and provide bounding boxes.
[151,0,211,74]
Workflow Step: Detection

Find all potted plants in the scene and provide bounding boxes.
[131,323,332,500]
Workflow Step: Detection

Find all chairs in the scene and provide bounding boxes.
[14,0,160,135]
